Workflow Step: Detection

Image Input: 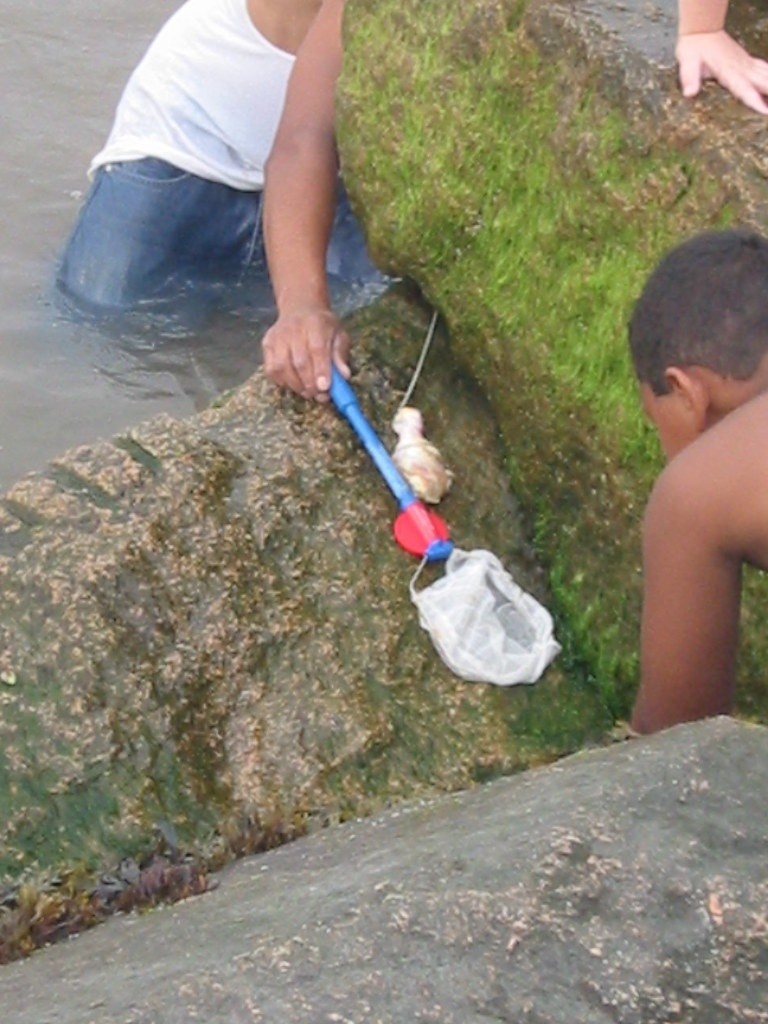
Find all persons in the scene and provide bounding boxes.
[627,230,768,737]
[59,0,768,404]
